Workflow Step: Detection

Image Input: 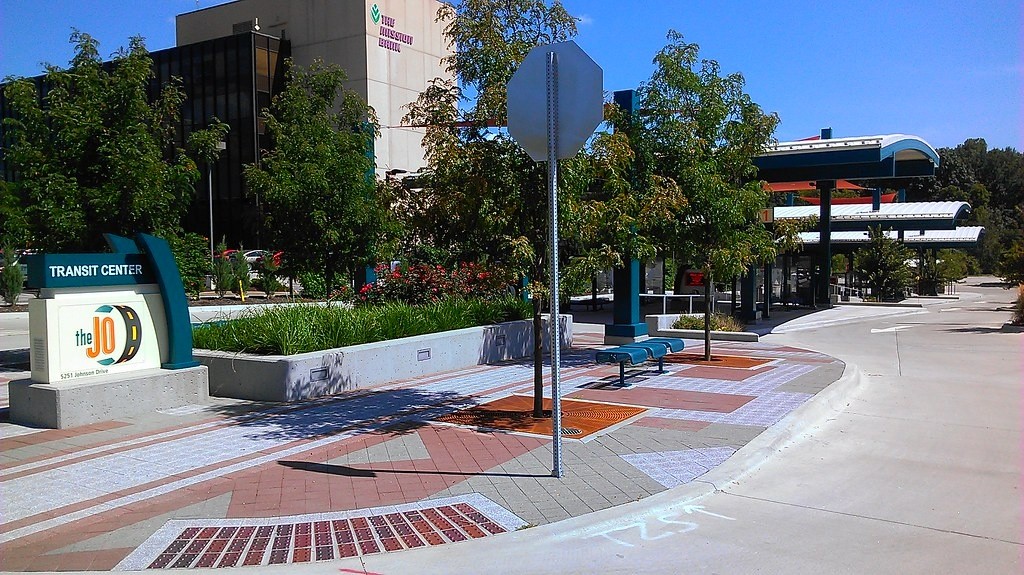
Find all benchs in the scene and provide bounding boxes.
[594,338,684,387]
[579,298,609,311]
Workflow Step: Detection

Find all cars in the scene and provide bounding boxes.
[257,252,283,270]
[231,249,269,272]
[214,250,240,261]
